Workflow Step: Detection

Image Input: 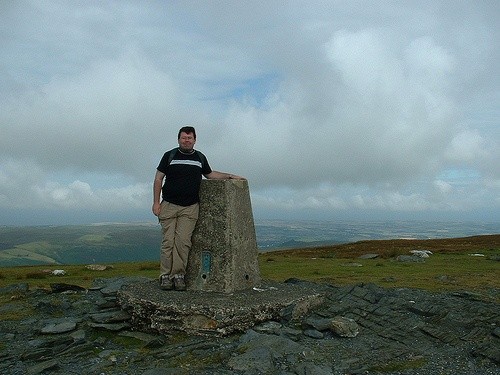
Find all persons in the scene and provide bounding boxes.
[152,125,247,292]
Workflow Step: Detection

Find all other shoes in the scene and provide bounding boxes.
[160,276,174,290]
[174,278,186,291]
